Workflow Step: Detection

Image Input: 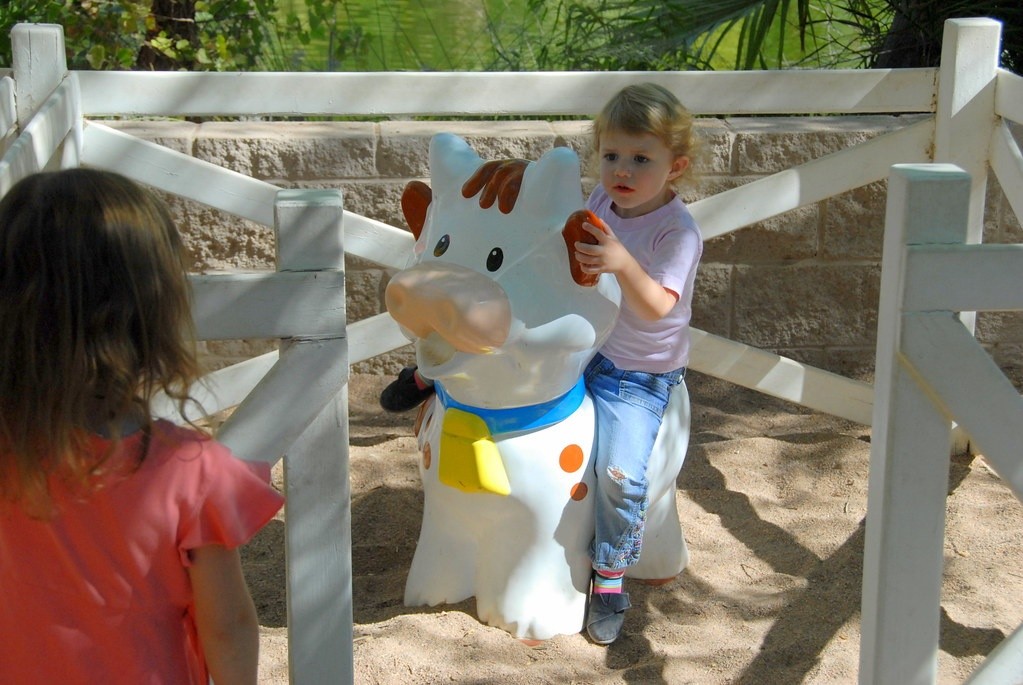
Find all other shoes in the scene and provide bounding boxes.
[379,366,435,413]
[586,568,631,644]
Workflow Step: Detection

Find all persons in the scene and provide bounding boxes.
[0,168,285,685]
[380,80,704,645]
